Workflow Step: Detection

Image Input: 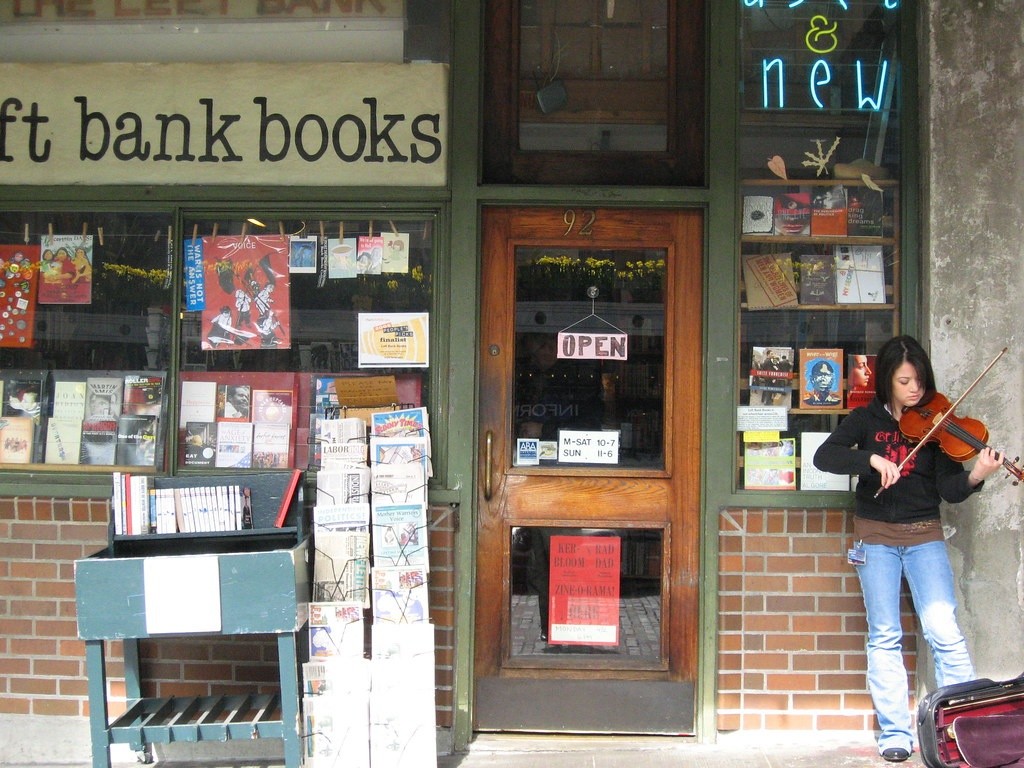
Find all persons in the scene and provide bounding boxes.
[814,335,1004,761]
[847,355,872,409]
[752,349,794,406]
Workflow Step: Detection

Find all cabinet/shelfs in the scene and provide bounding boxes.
[70,466,310,768]
[732,166,901,491]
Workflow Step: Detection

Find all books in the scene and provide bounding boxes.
[299,405,438,768]
[847,353,879,409]
[113,472,253,537]
[0,374,295,470]
[800,348,844,409]
[749,345,794,408]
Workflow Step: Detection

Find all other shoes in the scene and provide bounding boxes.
[883,748,908,761]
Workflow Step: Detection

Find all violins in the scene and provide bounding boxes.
[899,391,1024,486]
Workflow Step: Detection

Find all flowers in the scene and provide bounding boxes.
[383,264,432,303]
[100,262,169,296]
[532,253,667,293]
